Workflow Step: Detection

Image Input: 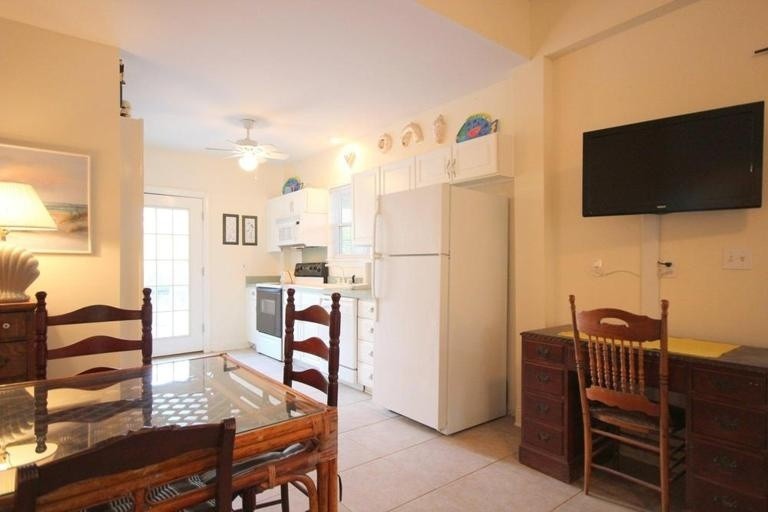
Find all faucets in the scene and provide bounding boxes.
[324,263,347,282]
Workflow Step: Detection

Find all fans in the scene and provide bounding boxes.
[205,119,278,152]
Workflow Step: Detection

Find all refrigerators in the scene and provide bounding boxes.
[369,182,508,436]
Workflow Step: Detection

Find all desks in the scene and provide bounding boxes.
[0,352,338,511]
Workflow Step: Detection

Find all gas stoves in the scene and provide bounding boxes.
[254,278,327,289]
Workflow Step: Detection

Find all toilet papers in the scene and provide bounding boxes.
[362,261,372,284]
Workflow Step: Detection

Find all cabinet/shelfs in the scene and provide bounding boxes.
[352,156,418,245]
[420,130,518,185]
[358,300,383,397]
[269,187,331,248]
[3,306,46,383]
[280,289,321,369]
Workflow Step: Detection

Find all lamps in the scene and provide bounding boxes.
[0,388,58,496]
[1,181,59,303]
[239,155,256,171]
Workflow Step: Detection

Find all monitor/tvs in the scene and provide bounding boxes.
[581,99,765,219]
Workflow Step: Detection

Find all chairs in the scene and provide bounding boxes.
[33,365,153,453]
[38,420,234,512]
[235,287,342,512]
[38,288,153,379]
[570,294,686,512]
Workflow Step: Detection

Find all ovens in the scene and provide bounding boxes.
[255,287,283,363]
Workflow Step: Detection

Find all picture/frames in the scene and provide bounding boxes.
[242,216,258,246]
[222,214,239,245]
[1,142,93,264]
